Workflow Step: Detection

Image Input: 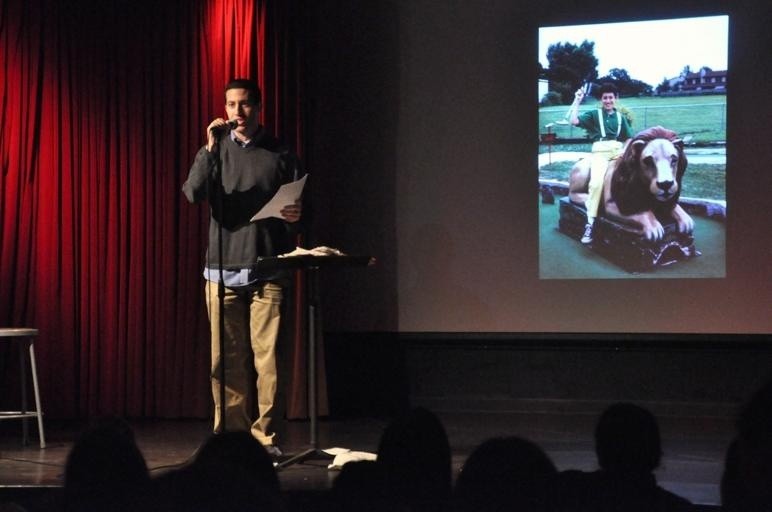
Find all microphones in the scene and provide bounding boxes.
[208,119,241,134]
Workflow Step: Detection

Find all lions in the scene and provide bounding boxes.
[568,125,696,243]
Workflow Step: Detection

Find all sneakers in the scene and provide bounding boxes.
[264,445,283,459]
[581,226,594,244]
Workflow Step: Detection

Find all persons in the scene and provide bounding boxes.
[55,384,771,512]
[564,79,640,247]
[180,77,313,459]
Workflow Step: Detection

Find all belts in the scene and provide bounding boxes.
[593,137,621,141]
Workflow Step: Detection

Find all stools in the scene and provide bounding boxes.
[0,328,47,449]
